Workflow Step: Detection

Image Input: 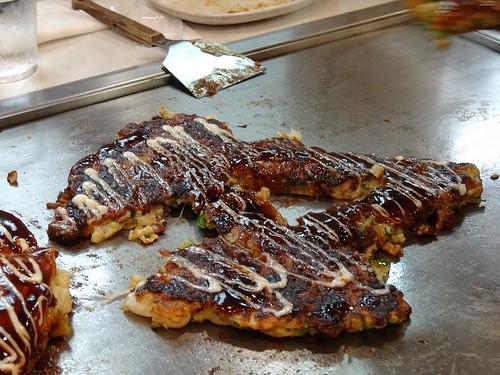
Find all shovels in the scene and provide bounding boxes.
[71,0,268,100]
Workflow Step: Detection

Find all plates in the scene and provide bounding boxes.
[152,0,314,24]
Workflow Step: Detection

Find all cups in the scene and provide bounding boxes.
[0,0,39,84]
